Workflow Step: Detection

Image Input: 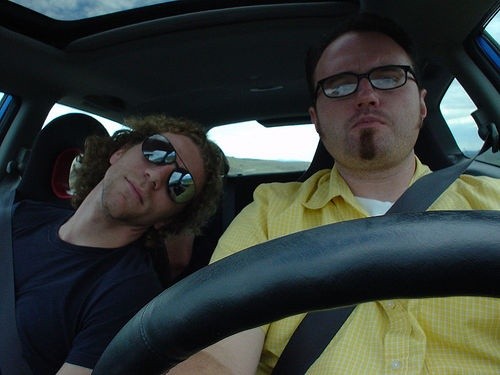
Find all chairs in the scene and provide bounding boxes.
[9,113,112,211]
[297,118,456,183]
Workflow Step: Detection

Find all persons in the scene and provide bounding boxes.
[0,112,231,375]
[161,24,500,375]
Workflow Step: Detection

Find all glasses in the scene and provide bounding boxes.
[313,64,422,109]
[141,133,197,204]
